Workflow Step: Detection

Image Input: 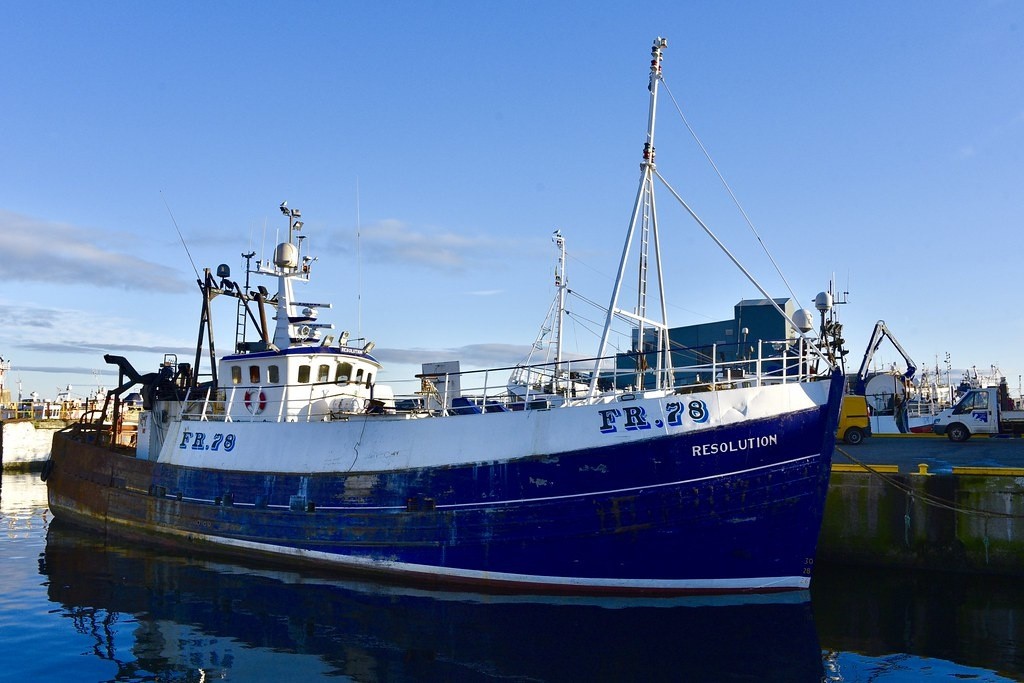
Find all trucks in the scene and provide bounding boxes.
[931,386,1024,442]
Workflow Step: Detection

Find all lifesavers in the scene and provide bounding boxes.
[245,386,266,415]
[40,459,53,481]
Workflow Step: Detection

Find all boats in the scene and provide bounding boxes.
[38,31,851,663]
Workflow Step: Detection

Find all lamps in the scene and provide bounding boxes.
[322,334,335,347]
[363,341,375,353]
[340,331,350,344]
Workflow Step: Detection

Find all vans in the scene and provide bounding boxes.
[835,395,872,445]
